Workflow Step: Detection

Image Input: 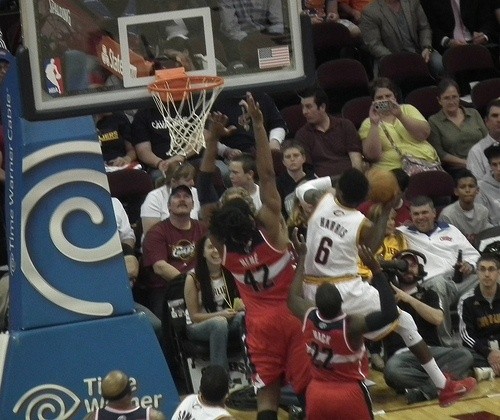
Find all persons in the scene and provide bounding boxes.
[29,0,500,420]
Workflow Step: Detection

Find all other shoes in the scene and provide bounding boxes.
[404,387,427,405]
[475,365,495,381]
[370,352,385,370]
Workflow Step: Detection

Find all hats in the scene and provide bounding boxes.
[167,184,194,202]
[485,142,500,160]
[395,252,418,265]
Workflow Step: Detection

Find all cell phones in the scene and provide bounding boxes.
[373,99,396,113]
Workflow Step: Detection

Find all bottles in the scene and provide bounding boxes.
[453,250,464,283]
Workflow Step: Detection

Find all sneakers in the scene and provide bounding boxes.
[438,379,477,406]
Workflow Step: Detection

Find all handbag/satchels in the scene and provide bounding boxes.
[403,153,443,178]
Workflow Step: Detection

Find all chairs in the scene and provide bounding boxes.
[109,169,153,226]
[341,96,373,130]
[319,61,369,113]
[378,52,426,98]
[443,44,492,97]
[472,78,500,116]
[166,279,247,396]
[405,86,442,119]
[280,105,308,139]
